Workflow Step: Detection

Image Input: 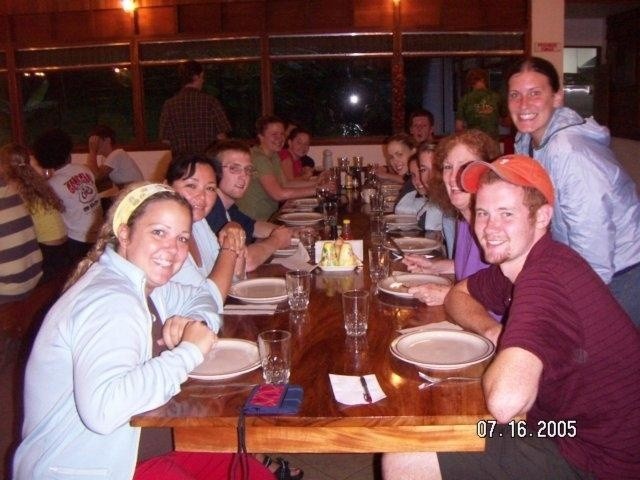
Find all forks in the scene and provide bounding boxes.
[418,368,481,386]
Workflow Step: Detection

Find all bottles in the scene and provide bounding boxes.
[317,149,378,194]
[323,196,352,240]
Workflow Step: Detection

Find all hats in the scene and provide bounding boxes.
[460,154,555,206]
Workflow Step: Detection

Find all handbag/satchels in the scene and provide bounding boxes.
[238,383,303,416]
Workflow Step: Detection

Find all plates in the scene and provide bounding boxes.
[275,211,325,227]
[272,247,301,256]
[389,328,496,372]
[293,197,319,207]
[376,274,454,300]
[226,276,291,304]
[185,336,272,382]
[380,214,422,227]
[381,237,442,256]
[314,239,364,272]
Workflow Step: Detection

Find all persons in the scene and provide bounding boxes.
[160,60,230,157]
[161,117,336,314]
[374,104,499,306]
[12,179,280,479]
[504,54,640,329]
[379,154,638,480]
[0,126,145,301]
[158,151,304,479]
[455,69,503,139]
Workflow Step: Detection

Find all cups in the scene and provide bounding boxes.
[342,289,369,338]
[258,330,292,384]
[368,190,390,282]
[286,269,312,313]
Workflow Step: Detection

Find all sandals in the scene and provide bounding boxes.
[262,455,303,478]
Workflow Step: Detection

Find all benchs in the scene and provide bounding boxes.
[0,263,72,364]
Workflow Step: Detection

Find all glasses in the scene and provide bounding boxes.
[219,163,254,176]
